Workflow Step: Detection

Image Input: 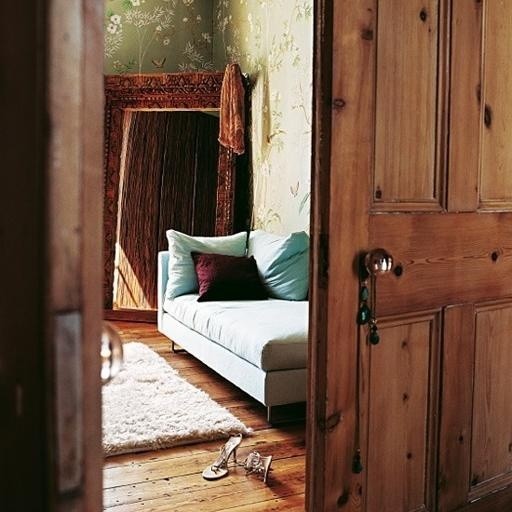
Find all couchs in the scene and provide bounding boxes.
[158,249,309,421]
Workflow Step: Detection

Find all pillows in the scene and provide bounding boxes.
[166,228,309,301]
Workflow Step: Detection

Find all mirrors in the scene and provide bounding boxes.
[103,72,236,323]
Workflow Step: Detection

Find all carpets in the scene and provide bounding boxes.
[102,341,258,459]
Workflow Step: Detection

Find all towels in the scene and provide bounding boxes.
[218,64,245,155]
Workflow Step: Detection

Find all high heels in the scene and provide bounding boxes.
[201,432,244,481]
[242,450,274,484]
